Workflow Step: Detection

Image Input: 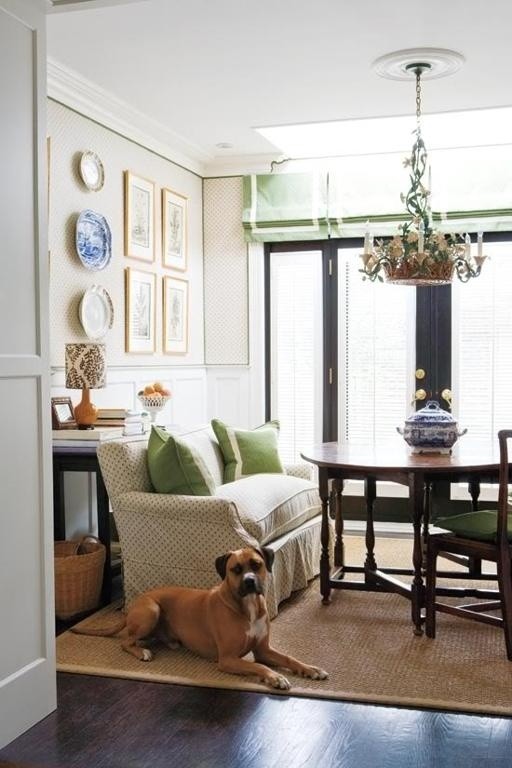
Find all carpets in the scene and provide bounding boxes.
[54,533,511,718]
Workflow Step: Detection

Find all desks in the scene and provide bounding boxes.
[53,445,113,608]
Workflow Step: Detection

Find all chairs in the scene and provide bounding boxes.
[424,430,512,662]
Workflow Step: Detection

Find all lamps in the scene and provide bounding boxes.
[358,48,486,287]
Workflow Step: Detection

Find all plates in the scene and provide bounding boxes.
[75,150,114,342]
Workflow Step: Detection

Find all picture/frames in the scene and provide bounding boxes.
[125,266,156,352]
[51,398,78,429]
[162,275,189,355]
[161,187,188,272]
[124,169,156,264]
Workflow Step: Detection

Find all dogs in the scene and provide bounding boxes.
[67,546,330,690]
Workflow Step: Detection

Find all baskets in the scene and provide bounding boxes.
[54,535,106,621]
[138,395,170,412]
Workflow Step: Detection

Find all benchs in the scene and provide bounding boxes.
[96,428,335,622]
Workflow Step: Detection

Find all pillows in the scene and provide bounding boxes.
[147,424,217,496]
[434,510,512,541]
[211,418,286,485]
